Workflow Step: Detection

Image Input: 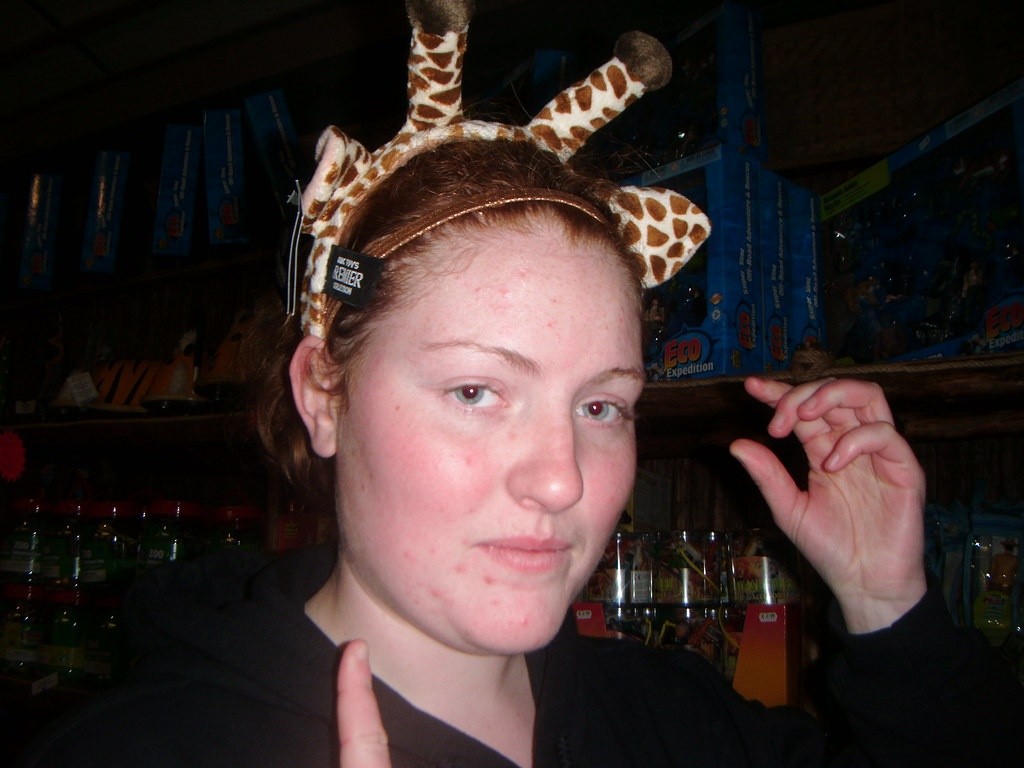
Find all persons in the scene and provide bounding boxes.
[8,1,1024,768]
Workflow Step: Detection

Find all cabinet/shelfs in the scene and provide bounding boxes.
[0,162,1024,465]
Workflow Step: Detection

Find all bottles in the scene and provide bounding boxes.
[0,496,258,654]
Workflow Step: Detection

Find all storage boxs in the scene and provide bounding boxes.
[760,1,1024,171]
[0,85,1024,709]
